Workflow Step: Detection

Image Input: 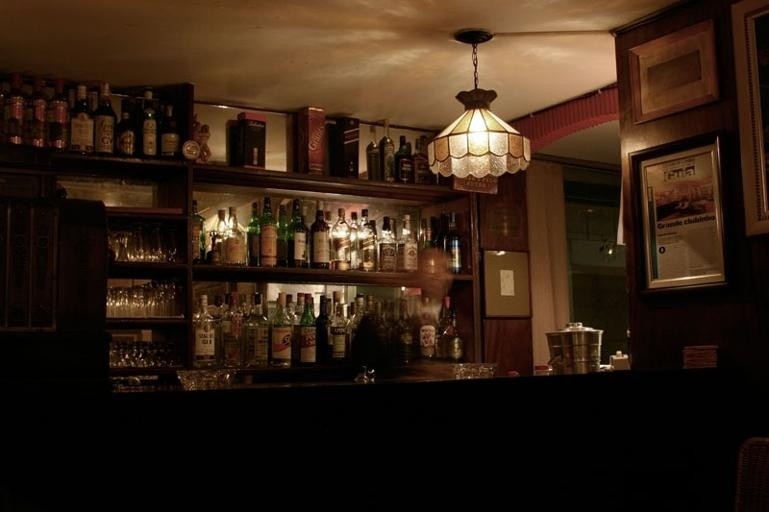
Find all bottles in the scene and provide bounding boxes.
[0,72,182,158]
[192,284,465,371]
[367,119,449,185]
[190,194,464,275]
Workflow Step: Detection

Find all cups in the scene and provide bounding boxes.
[104,223,183,317]
[110,338,187,368]
[452,361,497,379]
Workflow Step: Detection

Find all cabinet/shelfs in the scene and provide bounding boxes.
[4,142,475,372]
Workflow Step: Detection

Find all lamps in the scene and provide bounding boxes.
[427,27,533,177]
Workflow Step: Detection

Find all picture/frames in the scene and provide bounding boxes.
[728,2,769,237]
[625,16,718,127]
[481,247,532,319]
[628,127,736,303]
